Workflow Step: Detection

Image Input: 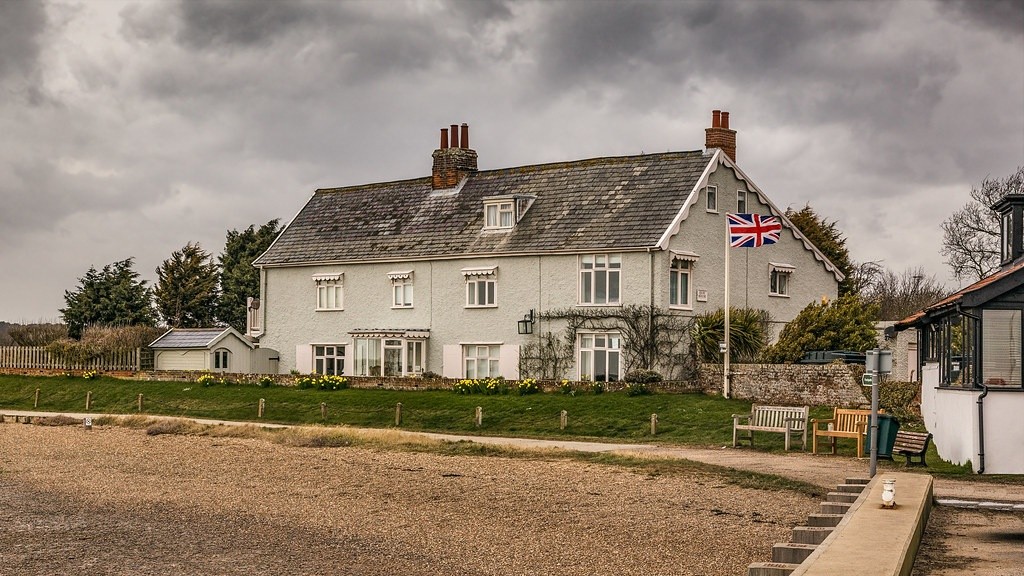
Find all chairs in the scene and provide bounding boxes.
[369,366,389,376]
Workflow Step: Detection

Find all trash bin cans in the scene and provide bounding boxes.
[865,414,901,461]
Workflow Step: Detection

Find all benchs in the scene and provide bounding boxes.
[892,430,933,468]
[810,407,886,459]
[731,402,810,451]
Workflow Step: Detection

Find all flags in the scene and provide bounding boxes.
[725,212,784,249]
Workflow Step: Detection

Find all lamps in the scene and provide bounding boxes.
[517,309,535,334]
